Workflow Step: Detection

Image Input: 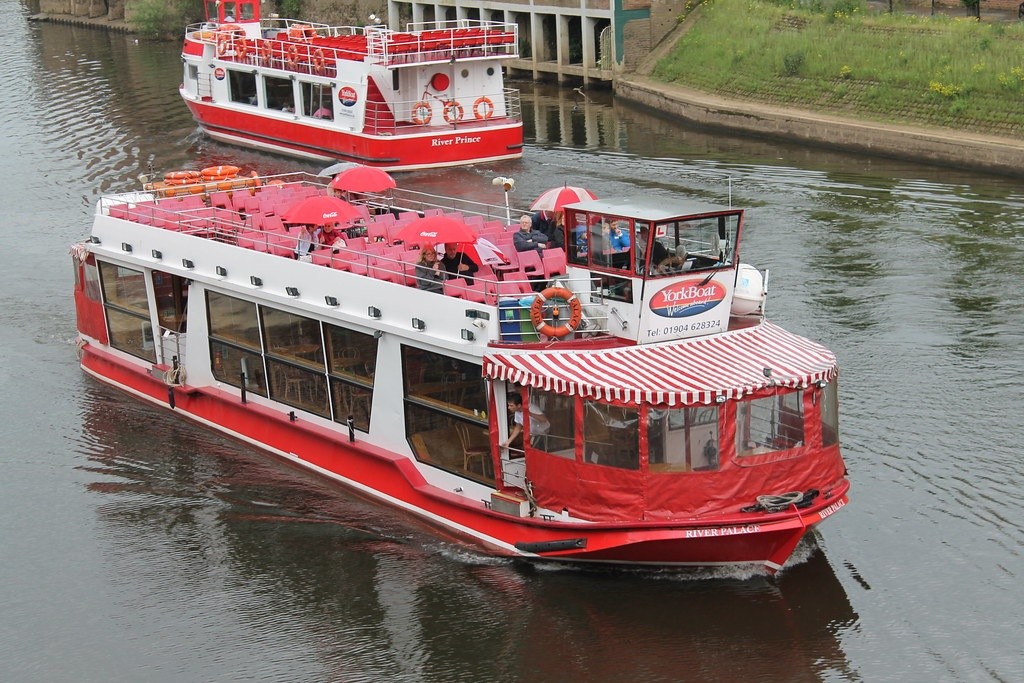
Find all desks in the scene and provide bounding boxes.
[281,343,320,356]
[412,381,481,395]
[163,314,186,328]
[334,357,363,368]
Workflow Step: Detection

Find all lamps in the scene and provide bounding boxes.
[91,236,100,243]
[286,285,299,297]
[216,266,227,276]
[250,276,262,286]
[183,259,193,267]
[461,329,473,340]
[412,319,425,330]
[121,243,132,251]
[368,307,382,318]
[152,249,163,260]
[324,296,339,307]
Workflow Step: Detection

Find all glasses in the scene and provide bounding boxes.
[425,251,435,256]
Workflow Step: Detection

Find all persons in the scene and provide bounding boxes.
[326,174,365,204]
[531,210,631,252]
[502,393,550,452]
[224,11,235,22]
[293,223,346,260]
[622,226,669,270]
[312,99,332,118]
[513,215,548,259]
[414,244,448,295]
[439,243,479,285]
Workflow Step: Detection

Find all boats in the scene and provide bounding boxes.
[69,164,850,572]
[178,0,523,172]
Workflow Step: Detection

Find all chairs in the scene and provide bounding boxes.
[108,185,632,306]
[227,27,515,75]
[271,335,492,481]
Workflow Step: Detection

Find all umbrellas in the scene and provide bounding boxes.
[529,181,597,213]
[316,162,396,192]
[280,192,361,237]
[394,214,478,265]
[433,236,511,278]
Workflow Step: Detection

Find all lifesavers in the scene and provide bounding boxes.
[531,288,583,336]
[201,166,238,179]
[288,45,298,66]
[217,37,226,54]
[289,24,318,43]
[216,24,245,38]
[444,101,464,123]
[262,43,271,61]
[474,97,493,119]
[313,49,325,72]
[236,38,246,59]
[251,170,260,186]
[412,102,432,124]
[164,171,201,185]
